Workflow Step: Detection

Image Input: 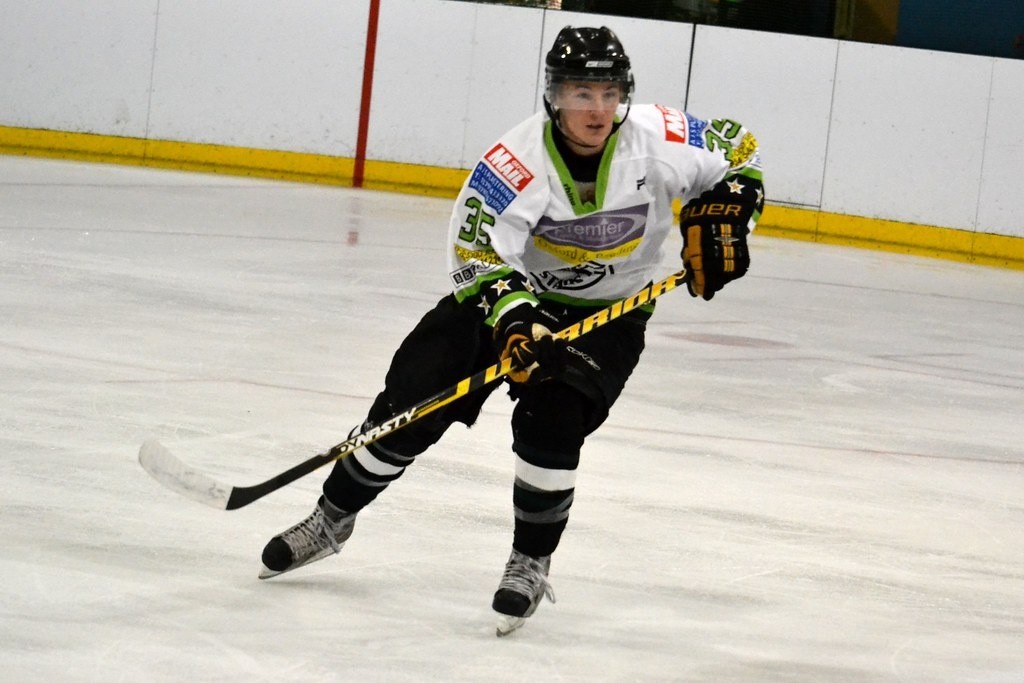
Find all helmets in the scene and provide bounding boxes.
[544,25,631,104]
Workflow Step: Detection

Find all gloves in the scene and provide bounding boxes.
[678,189,754,301]
[492,302,569,395]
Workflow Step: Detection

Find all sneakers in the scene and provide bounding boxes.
[258,494,355,578]
[491,547,556,637]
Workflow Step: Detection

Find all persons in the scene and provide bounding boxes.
[256,26,762,643]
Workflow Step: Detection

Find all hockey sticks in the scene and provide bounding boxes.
[140,268,688,515]
